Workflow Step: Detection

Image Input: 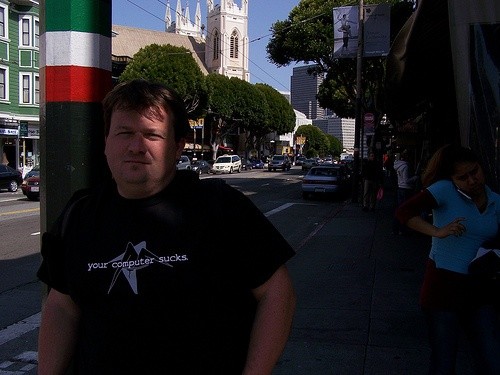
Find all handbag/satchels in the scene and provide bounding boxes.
[377,186,383,201]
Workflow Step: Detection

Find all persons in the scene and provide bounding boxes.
[394,145,500,375]
[362,148,420,237]
[422,145,463,220]
[36,80,297,375]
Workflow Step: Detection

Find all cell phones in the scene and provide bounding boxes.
[453,183,473,200]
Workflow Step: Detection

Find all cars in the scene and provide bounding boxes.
[191,160,211,175]
[0,164,23,192]
[175,156,192,170]
[295,156,354,201]
[251,159,264,169]
[208,160,215,164]
[268,155,292,172]
[242,159,252,170]
[24,165,40,179]
[20,176,41,200]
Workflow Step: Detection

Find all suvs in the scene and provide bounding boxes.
[211,155,242,174]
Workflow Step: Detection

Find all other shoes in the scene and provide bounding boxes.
[362,207,369,211]
[375,210,380,216]
